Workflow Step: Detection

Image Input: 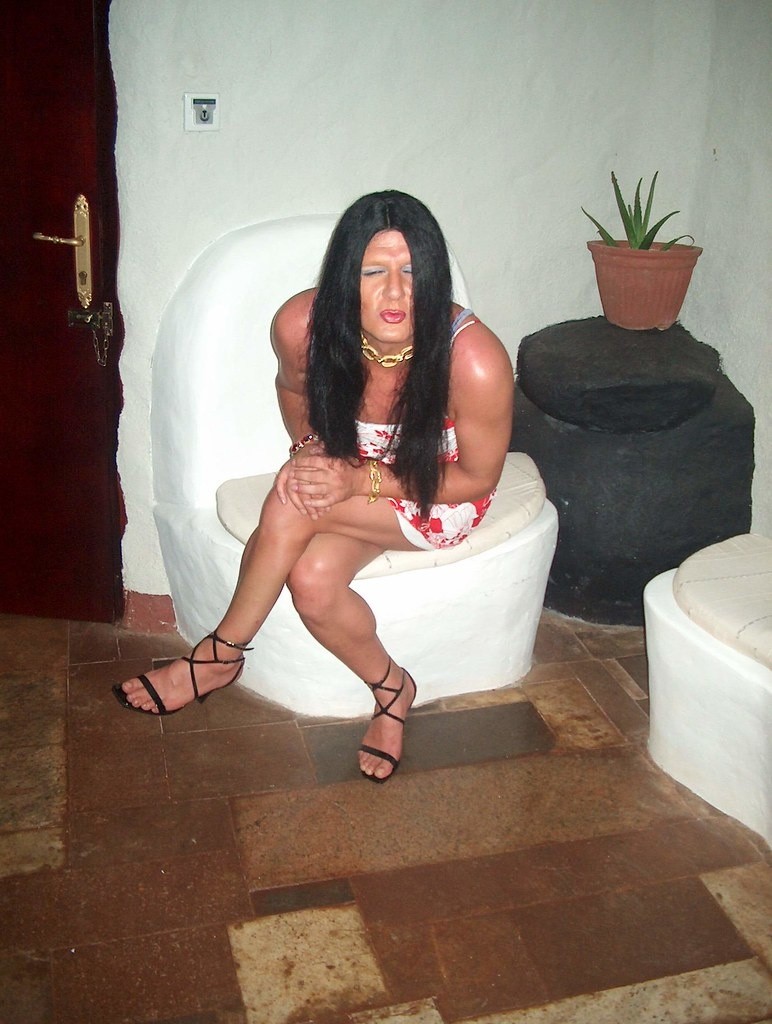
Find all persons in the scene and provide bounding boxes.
[112,191,516,786]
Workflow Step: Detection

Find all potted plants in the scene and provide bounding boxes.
[581,171,706,333]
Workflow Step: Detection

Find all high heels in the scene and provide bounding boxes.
[353,658,418,788]
[114,628,252,716]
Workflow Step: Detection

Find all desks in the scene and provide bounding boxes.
[510,317,756,627]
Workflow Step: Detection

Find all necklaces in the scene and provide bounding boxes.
[360,332,413,367]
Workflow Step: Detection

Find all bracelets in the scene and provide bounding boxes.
[289,431,320,458]
[368,460,383,504]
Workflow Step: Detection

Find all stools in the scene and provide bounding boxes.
[642,529,772,847]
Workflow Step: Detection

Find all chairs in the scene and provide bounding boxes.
[151,212,559,718]
